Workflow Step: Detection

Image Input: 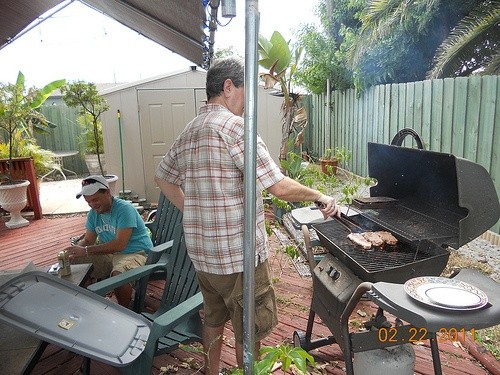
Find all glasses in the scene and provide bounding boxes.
[82,178,108,188]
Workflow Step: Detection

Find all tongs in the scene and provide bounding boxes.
[317,202,372,234]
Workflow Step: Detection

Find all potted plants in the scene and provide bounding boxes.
[57,80,120,196]
[0,79,45,229]
[272,139,306,224]
[318,146,339,176]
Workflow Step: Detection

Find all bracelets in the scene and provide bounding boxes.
[85,246,89,258]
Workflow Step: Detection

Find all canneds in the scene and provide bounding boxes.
[57,250,71,276]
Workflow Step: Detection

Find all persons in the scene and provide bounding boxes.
[63,175,153,308]
[153,58,341,375]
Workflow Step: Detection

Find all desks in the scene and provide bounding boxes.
[0,263,94,375]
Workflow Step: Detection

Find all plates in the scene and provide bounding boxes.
[403,275,488,312]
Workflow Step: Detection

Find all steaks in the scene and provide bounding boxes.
[346,229,398,248]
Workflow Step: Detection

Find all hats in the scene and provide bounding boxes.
[76,175,109,199]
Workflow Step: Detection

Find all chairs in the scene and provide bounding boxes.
[82,189,204,375]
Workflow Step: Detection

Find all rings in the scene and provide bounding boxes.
[331,205,336,208]
